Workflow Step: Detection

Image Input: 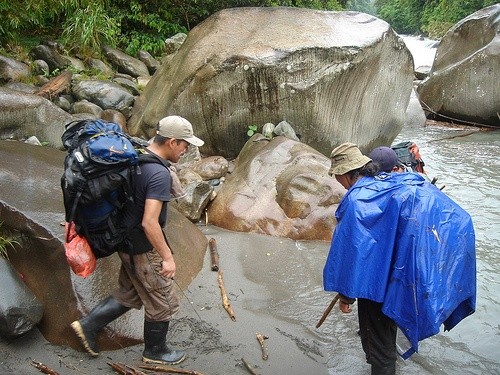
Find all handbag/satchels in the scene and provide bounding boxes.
[63,219,96,279]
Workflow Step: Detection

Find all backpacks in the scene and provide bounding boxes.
[389,140,425,174]
[61,117,171,260]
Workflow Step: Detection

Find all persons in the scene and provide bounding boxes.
[369,146,418,175]
[70,115,205,366]
[328,143,399,375]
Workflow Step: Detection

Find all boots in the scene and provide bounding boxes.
[141,318,188,365]
[70,296,132,357]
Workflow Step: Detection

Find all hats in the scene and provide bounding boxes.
[156,115,204,148]
[368,146,398,172]
[328,144,373,176]
